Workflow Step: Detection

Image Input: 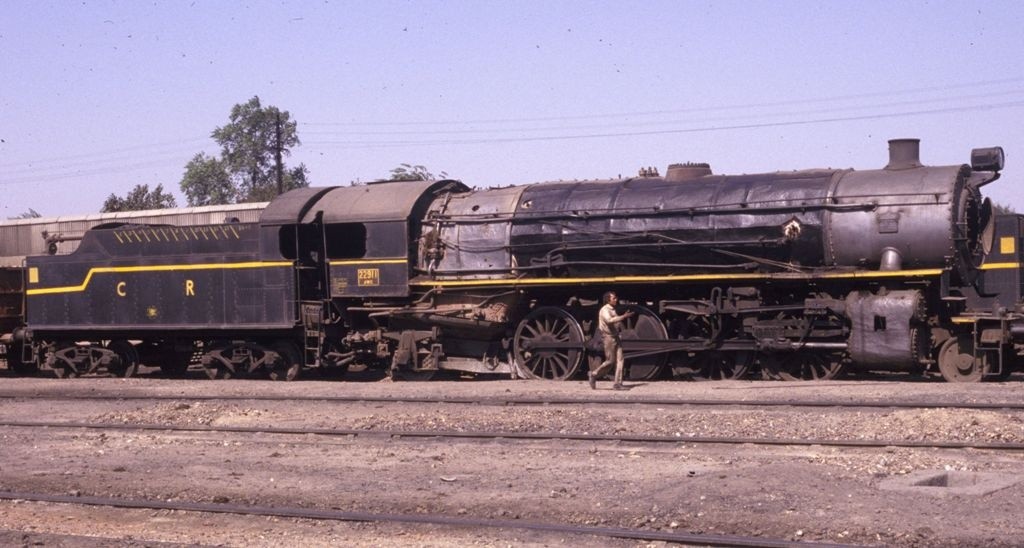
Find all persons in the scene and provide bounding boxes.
[587,291,633,390]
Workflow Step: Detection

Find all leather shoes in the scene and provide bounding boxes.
[613,384,630,390]
[587,371,596,389]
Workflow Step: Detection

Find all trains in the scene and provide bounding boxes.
[0,138,1024,383]
